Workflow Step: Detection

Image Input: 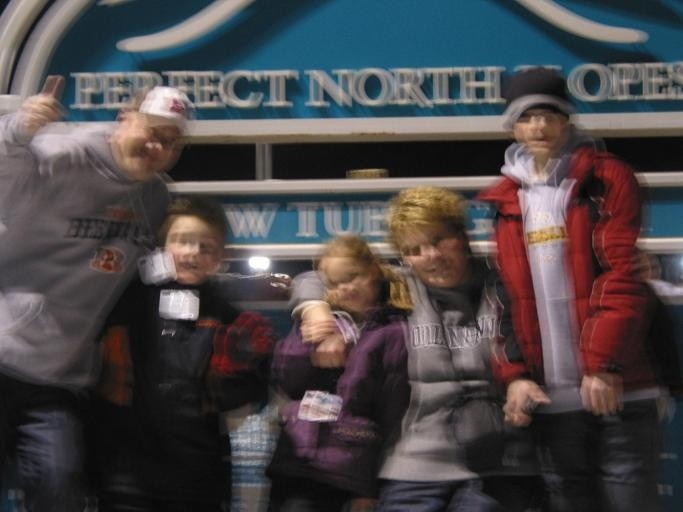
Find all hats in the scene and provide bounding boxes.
[120,84,196,142]
[501,69,575,129]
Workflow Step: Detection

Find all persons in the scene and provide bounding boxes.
[0,69,661,511]
